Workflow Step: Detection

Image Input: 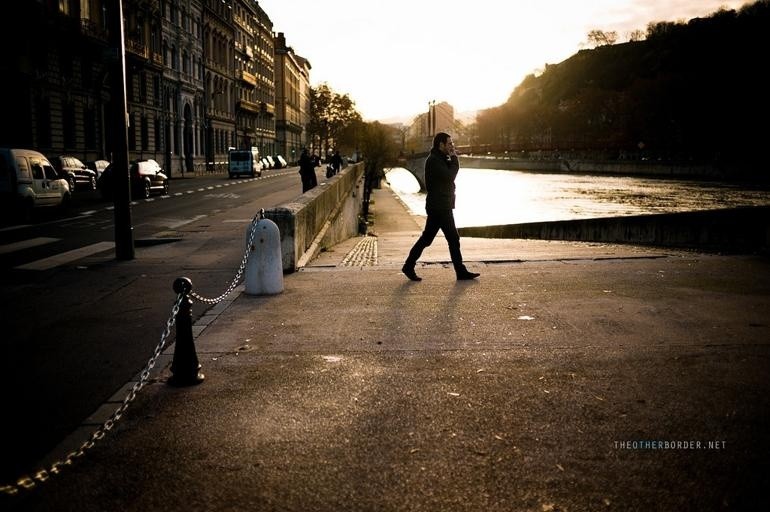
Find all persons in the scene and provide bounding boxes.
[401,132,481,282]
[329,151,343,175]
[342,154,349,170]
[299,147,318,193]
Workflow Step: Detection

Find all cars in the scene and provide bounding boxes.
[0,148,168,223]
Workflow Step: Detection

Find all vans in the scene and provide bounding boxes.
[227,146,262,178]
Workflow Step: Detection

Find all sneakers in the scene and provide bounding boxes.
[402,264,481,281]
